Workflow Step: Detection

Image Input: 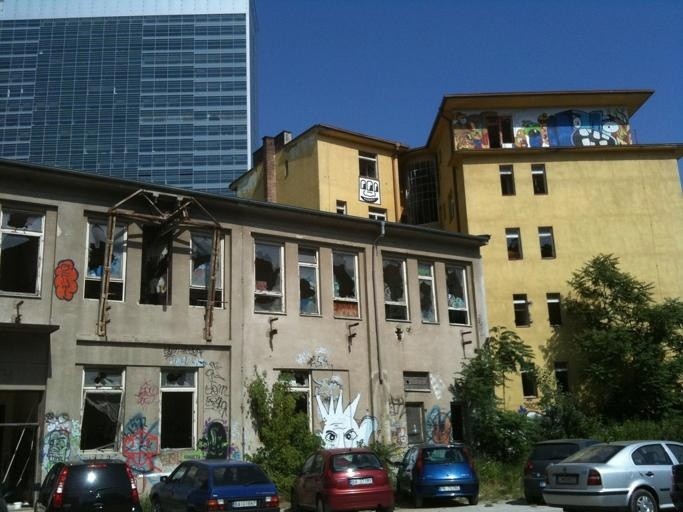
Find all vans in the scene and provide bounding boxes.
[29,460,141,512]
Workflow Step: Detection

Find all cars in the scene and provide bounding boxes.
[149,458,280,510]
[396,441,482,505]
[521,438,599,504]
[288,446,396,512]
[669,463,682,512]
[543,441,682,512]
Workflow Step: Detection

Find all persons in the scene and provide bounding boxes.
[147,271,167,304]
